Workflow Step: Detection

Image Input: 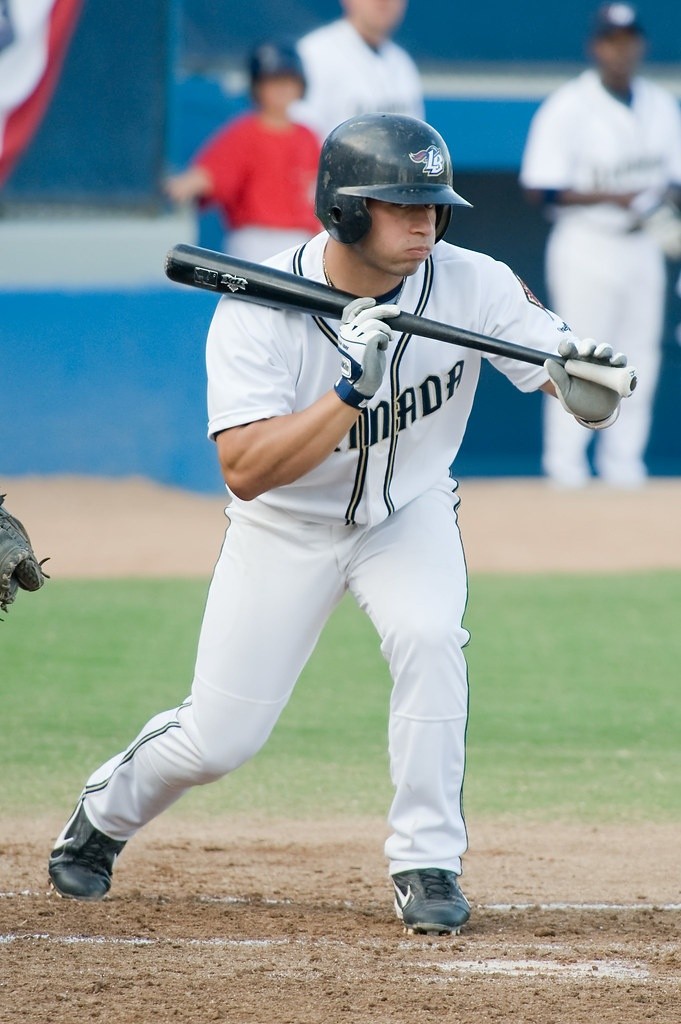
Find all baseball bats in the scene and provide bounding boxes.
[164,241,639,400]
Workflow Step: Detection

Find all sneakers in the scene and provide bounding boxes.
[48,798,128,903]
[392,869,472,935]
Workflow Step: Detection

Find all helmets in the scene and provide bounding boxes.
[314,115,474,245]
[249,41,306,101]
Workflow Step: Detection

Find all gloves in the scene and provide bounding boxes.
[543,340,626,430]
[331,296,401,412]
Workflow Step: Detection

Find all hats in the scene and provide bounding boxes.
[588,6,647,36]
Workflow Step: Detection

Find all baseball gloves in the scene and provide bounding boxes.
[0,491,53,622]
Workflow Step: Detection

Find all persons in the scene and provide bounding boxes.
[47,114,625,931]
[517,3,681,481]
[277,1,427,142]
[161,39,330,270]
